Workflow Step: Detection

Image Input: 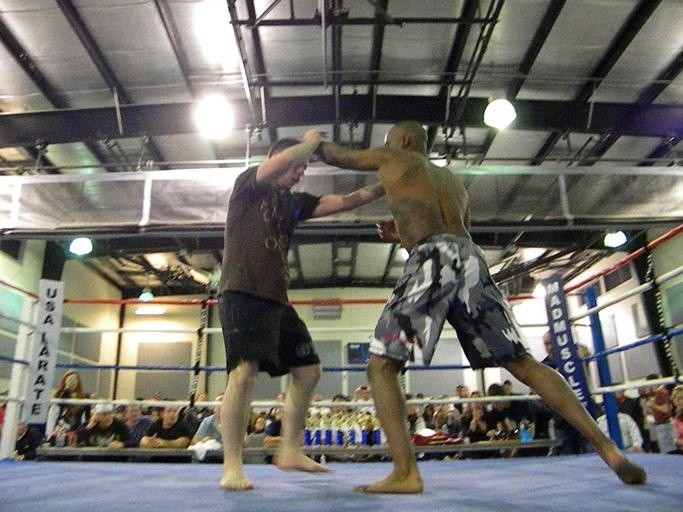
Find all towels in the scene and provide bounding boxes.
[187,439,221,461]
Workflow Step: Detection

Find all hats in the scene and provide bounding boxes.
[94,403,114,414]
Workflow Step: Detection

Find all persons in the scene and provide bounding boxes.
[211,126,391,492]
[406,380,556,460]
[307,116,651,496]
[600,374,682,455]
[303,385,393,464]
[242,393,285,464]
[0,367,249,463]
[529,329,605,459]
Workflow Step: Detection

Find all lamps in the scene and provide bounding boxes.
[482,93,518,130]
[604,227,627,247]
[138,286,153,301]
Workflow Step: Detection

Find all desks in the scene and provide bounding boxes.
[36,438,565,463]
